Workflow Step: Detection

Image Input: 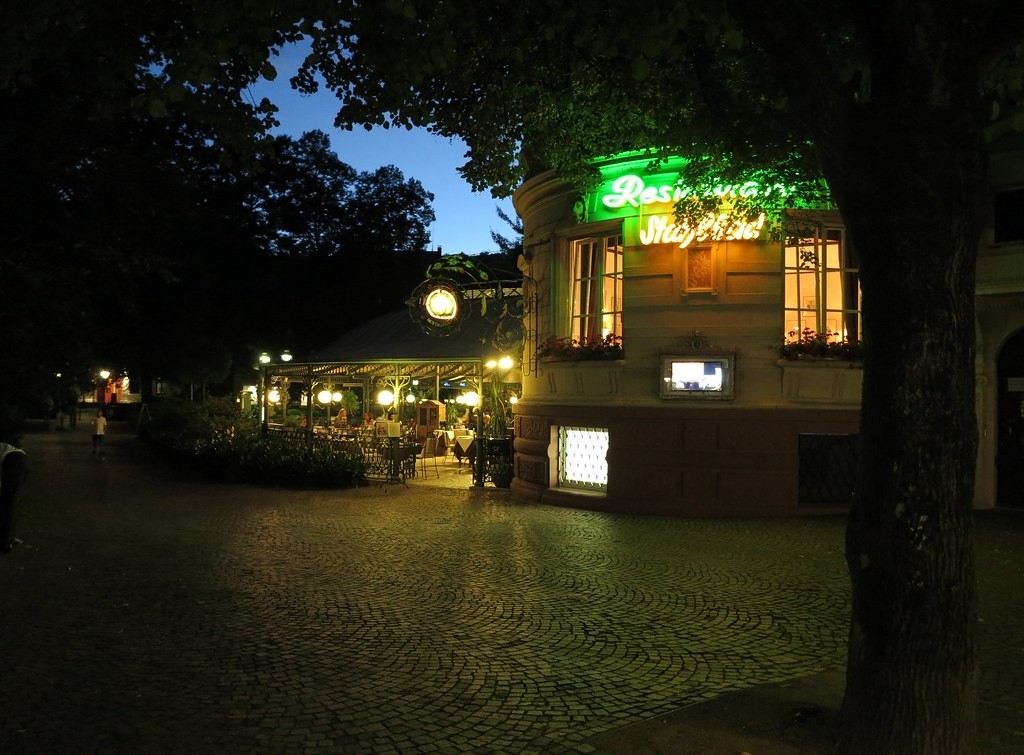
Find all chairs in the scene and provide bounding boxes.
[441,432,456,465]
[415,438,440,480]
[362,444,377,456]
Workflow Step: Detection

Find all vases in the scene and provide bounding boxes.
[544,353,573,363]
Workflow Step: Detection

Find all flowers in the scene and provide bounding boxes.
[767,327,863,369]
[531,334,624,364]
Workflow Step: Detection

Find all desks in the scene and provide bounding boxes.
[312,425,476,474]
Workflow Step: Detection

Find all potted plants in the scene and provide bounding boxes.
[484,368,514,488]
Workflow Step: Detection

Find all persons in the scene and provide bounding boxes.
[300,417,308,429]
[261,420,268,435]
[362,412,416,435]
[91,410,107,454]
